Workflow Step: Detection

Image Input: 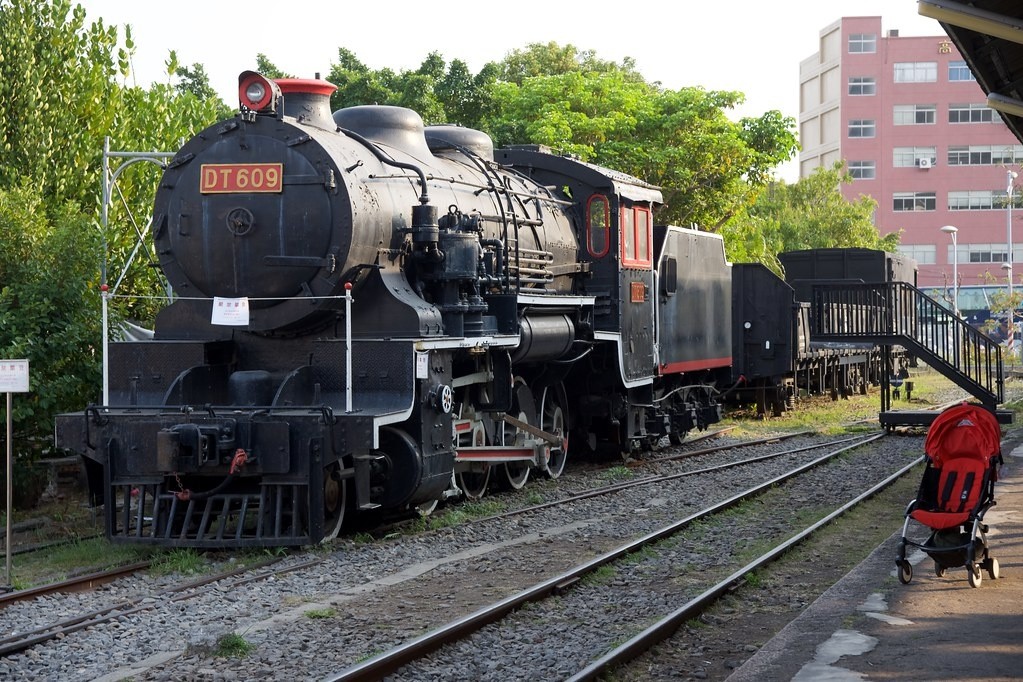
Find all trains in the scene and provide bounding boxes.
[54,70,919,551]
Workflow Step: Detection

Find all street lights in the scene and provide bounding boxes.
[1001,171,1018,352]
[940,226,958,316]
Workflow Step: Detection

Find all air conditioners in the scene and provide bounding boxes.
[919,157,932,169]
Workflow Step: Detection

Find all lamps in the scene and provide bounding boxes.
[905,381,913,400]
[890,380,903,400]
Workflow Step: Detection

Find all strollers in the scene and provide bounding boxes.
[896,399,1002,588]
